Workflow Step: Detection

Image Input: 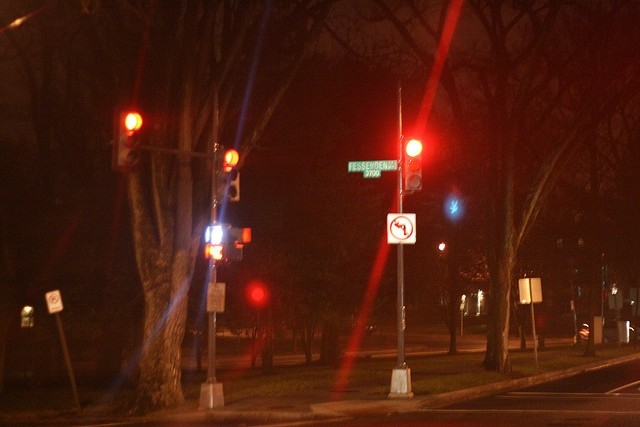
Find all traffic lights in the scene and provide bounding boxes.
[404,139,423,192]
[442,183,464,222]
[117,111,143,167]
[215,145,240,201]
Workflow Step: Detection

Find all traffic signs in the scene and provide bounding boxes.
[348,160,397,178]
